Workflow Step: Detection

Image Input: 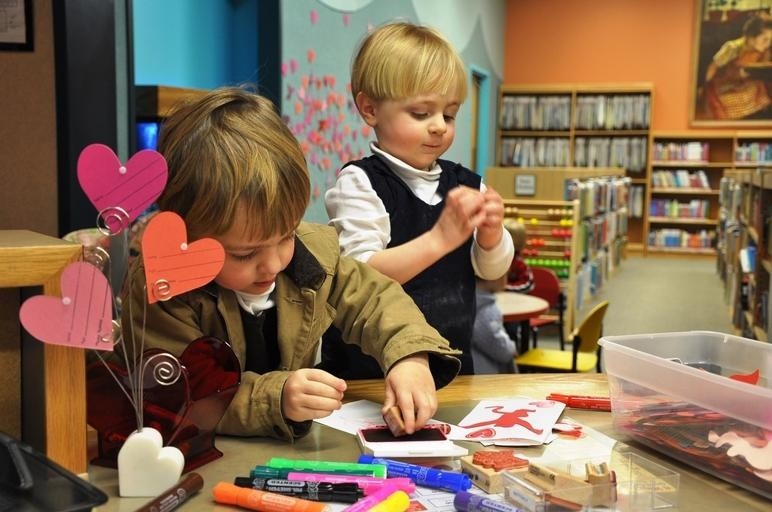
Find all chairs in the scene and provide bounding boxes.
[514,304,614,377]
[507,266,568,352]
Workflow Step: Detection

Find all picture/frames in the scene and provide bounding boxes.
[1,1,36,52]
[688,0,771,129]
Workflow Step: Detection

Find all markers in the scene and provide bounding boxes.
[547,394,611,412]
[363,424,451,434]
[211,456,521,512]
[140,472,204,512]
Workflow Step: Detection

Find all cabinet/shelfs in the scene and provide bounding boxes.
[646,133,736,252]
[499,84,570,167]
[570,85,655,256]
[713,166,772,346]
[736,134,772,167]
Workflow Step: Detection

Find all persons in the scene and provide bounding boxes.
[470,270,527,372]
[107,86,463,440]
[698,12,771,118]
[322,13,513,383]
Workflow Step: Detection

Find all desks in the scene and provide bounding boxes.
[494,288,551,355]
[53,368,772,511]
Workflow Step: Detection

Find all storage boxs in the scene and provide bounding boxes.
[598,327,772,502]
